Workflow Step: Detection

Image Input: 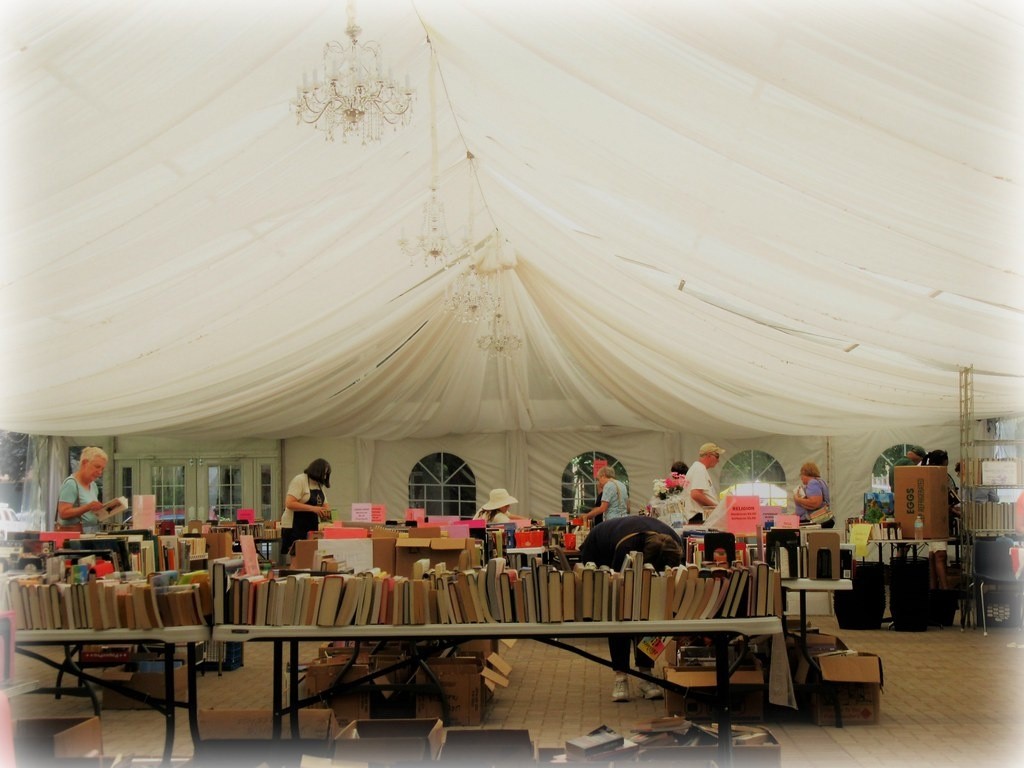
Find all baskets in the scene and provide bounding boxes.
[927,588,957,626]
[834,562,886,630]
[889,556,928,632]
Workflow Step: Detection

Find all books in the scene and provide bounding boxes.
[0,521,864,768]
[960,455,1024,623]
[93,496,128,522]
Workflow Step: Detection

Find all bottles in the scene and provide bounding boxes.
[914,516,923,541]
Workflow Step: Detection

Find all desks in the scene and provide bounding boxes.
[782,578,853,657]
[506,546,546,570]
[15,625,209,768]
[868,537,957,633]
[211,616,783,768]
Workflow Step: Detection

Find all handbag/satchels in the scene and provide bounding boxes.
[804,479,834,524]
[53,524,83,534]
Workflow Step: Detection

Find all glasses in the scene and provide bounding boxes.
[706,453,719,459]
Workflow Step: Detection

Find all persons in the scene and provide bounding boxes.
[794,462,837,528]
[578,516,685,700]
[281,458,332,565]
[684,442,726,525]
[58,446,108,533]
[473,488,525,523]
[584,467,630,526]
[888,445,1023,589]
[671,462,689,479]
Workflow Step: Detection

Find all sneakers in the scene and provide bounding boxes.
[612,676,629,702]
[636,677,663,699]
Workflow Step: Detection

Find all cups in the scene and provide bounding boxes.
[565,534,576,549]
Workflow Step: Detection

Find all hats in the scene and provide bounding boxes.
[482,489,518,510]
[699,442,726,456]
[910,446,925,458]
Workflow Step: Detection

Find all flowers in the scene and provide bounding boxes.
[652,471,691,501]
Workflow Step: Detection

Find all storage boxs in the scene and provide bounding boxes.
[662,617,884,726]
[894,465,949,540]
[514,530,544,548]
[295,525,476,576]
[637,725,780,768]
[12,637,638,768]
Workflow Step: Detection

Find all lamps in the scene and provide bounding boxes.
[289,0,524,363]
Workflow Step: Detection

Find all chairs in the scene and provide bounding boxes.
[968,536,1024,636]
